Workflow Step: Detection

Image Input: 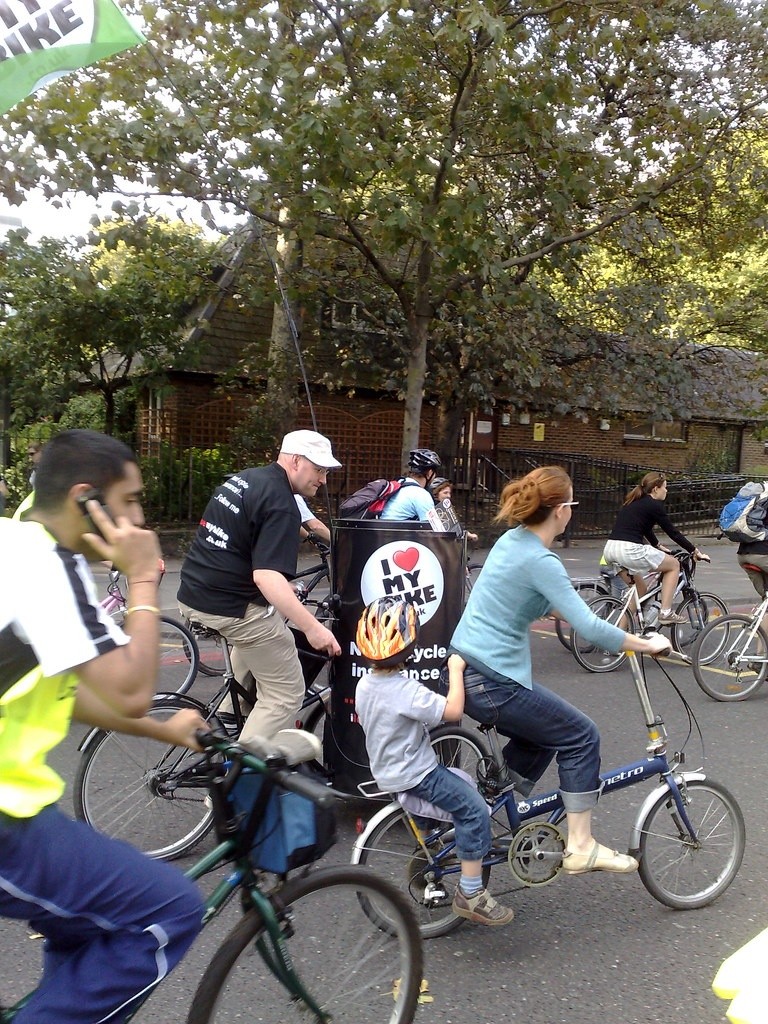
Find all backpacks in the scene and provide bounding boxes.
[719,480,768,542]
[338,476,420,519]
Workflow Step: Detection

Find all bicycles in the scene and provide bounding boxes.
[554,548,729,673]
[73,534,489,862]
[693,563,768,701]
[348,625,746,941]
[0,727,422,1023]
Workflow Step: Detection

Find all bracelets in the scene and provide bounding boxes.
[127,606,161,615]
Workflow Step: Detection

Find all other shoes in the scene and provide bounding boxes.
[204,794,214,809]
[562,840,639,875]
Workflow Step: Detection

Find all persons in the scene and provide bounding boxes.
[0,428,214,1024]
[440,465,673,875]
[12,468,166,573]
[736,477,768,683]
[293,493,331,542]
[429,477,474,577]
[378,448,447,533]
[599,471,710,632]
[354,597,515,926]
[175,430,341,812]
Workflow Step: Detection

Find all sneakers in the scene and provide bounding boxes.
[605,649,621,657]
[452,883,514,926]
[658,611,689,624]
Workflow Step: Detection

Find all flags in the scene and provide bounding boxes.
[0,0,148,119]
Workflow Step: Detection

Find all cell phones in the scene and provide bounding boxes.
[76,489,116,538]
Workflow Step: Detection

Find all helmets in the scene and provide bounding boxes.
[429,477,449,490]
[408,448,442,468]
[357,596,419,667]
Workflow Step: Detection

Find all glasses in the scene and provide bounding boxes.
[548,500,580,514]
[660,474,663,479]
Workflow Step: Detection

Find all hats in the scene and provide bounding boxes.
[280,430,341,468]
